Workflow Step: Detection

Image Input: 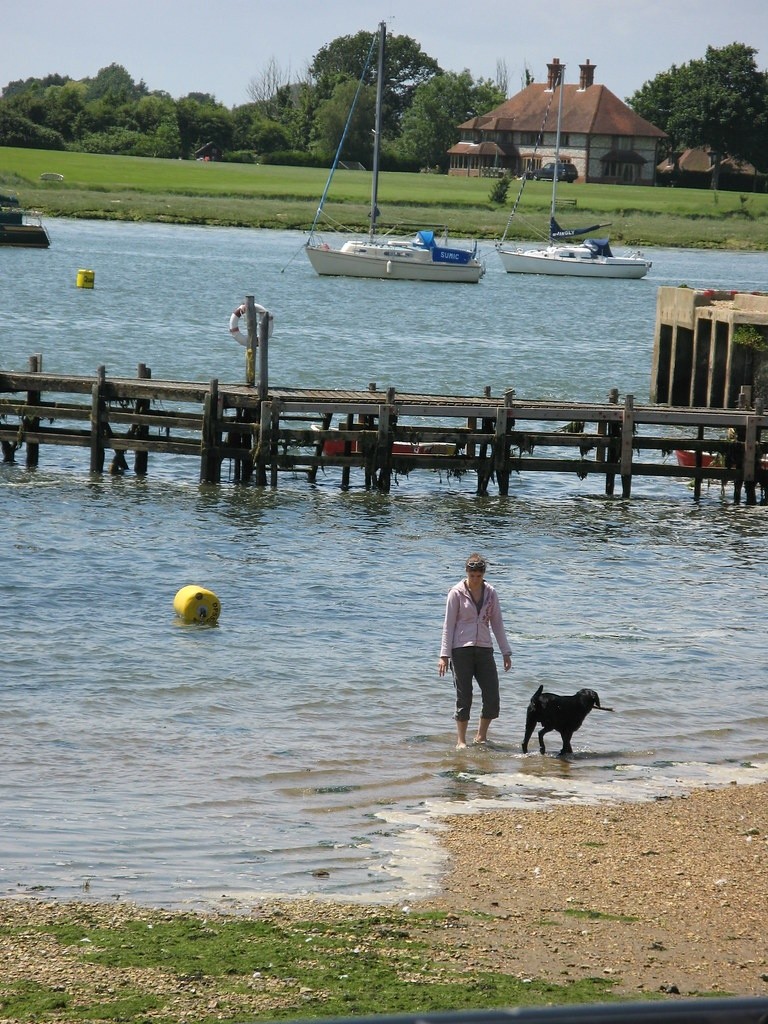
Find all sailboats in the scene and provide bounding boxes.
[305,20,482,281]
[496,64,654,277]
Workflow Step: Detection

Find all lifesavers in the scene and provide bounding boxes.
[226,300,276,350]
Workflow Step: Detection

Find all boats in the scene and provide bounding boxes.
[311,424,456,454]
[676,448,768,471]
[0,205,54,249]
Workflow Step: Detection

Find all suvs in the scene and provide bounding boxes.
[532,162,578,184]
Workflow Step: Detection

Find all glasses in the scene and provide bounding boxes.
[466,562,485,567]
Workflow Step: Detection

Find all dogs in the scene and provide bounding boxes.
[521,685,600,757]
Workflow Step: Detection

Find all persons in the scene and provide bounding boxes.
[438,552,512,750]
[196,150,212,161]
